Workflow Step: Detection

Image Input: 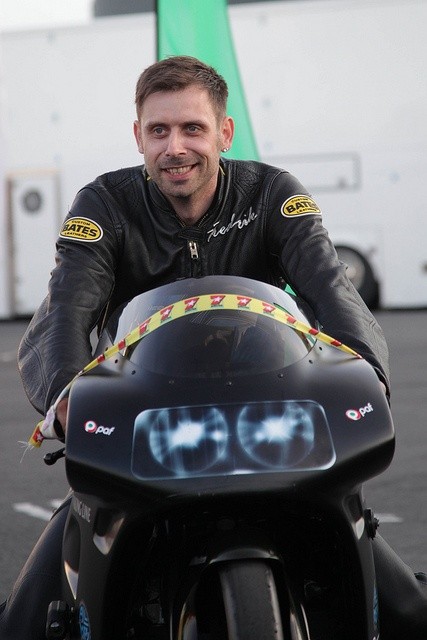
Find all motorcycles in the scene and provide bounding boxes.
[43,274,395,640]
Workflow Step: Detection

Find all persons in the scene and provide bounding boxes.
[0,56,427,640]
